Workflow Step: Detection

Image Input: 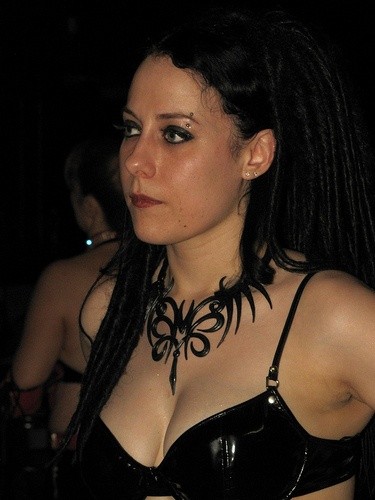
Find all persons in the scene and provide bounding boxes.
[0,0,375,500]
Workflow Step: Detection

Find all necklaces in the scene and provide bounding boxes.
[92,230,116,246]
[146,245,275,396]
